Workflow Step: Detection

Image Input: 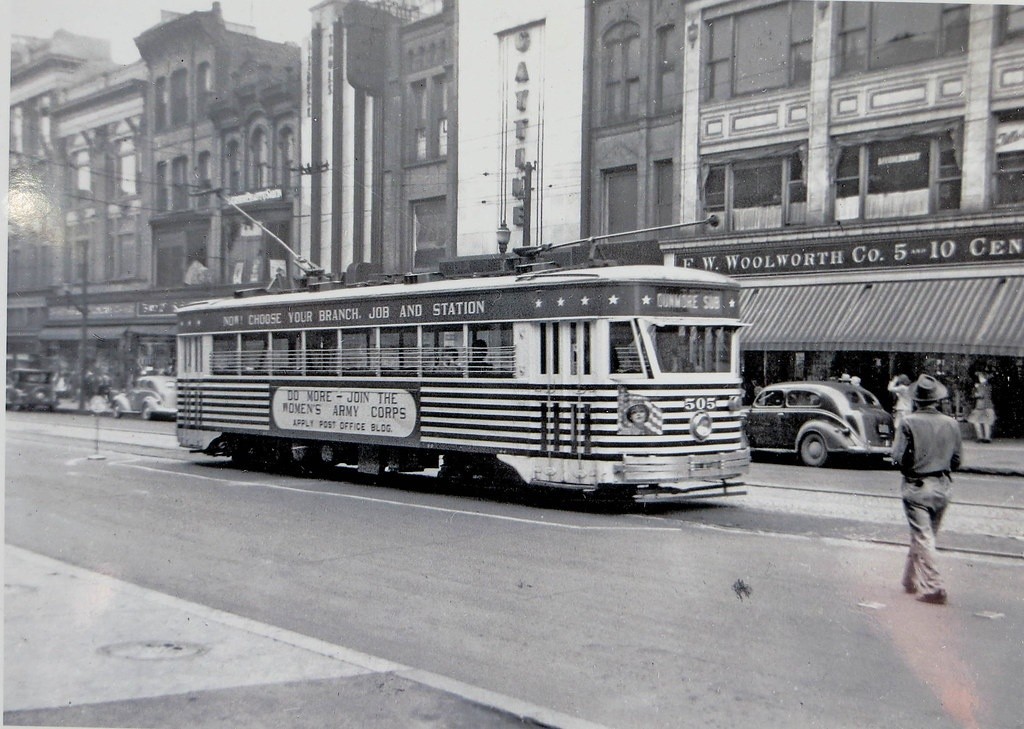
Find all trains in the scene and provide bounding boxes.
[175,189,750,510]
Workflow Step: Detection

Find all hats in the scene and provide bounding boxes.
[907,373,948,401]
[625,399,649,413]
[838,374,852,381]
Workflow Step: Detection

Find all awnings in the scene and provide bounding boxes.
[733,275,1024,361]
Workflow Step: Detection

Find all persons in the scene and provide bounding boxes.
[51,354,114,407]
[433,326,701,376]
[887,374,915,434]
[887,374,963,605]
[741,373,861,406]
[966,370,998,443]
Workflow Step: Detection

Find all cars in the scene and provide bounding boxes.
[741,380,896,467]
[109,376,179,420]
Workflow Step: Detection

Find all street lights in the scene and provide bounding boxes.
[493,221,512,272]
[57,236,90,413]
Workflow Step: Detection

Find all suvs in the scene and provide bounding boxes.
[7,368,58,412]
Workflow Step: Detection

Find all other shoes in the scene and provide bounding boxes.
[904,585,919,594]
[914,588,946,604]
